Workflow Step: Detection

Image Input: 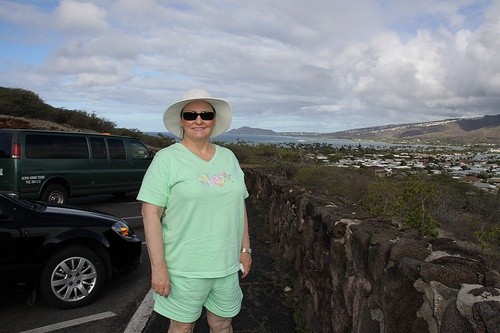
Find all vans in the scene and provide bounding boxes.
[0,127,159,206]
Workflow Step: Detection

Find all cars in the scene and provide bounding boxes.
[0,190,144,311]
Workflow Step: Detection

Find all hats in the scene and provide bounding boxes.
[163,89,232,140]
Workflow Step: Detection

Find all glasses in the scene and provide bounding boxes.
[182,111,215,121]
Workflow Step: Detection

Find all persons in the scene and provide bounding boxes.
[136,89,252,333]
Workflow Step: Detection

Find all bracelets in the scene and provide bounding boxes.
[241,247,252,254]
